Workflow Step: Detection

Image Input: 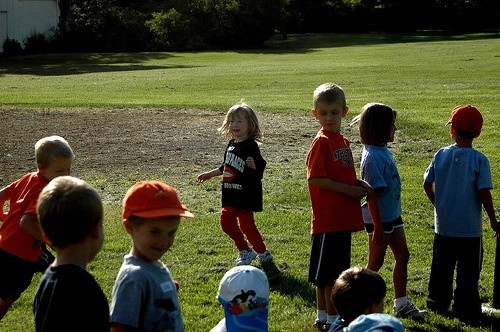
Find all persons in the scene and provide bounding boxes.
[304,80,376,332]
[1,135,76,323]
[326,267,406,332]
[208,264,271,332]
[196,102,275,266]
[359,103,431,322]
[422,102,500,321]
[33,174,111,332]
[108,178,195,332]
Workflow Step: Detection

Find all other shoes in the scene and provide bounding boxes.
[313,319,331,332]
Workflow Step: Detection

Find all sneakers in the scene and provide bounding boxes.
[257,250,274,263]
[234,248,257,266]
[393,300,427,322]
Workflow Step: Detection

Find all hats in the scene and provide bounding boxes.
[122,180,194,220]
[445,104,483,133]
[215,265,270,332]
